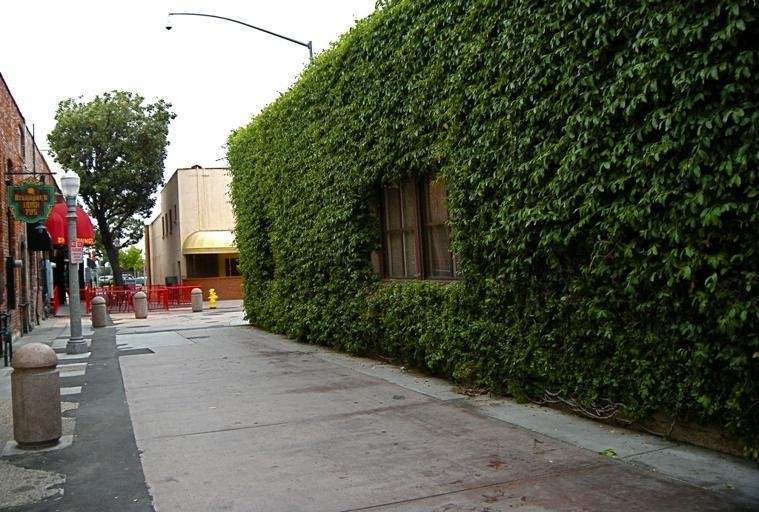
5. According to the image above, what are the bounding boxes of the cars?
[98,273,145,285]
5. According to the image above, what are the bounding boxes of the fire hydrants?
[208,287,218,309]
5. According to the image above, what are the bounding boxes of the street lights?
[60,168,87,354]
